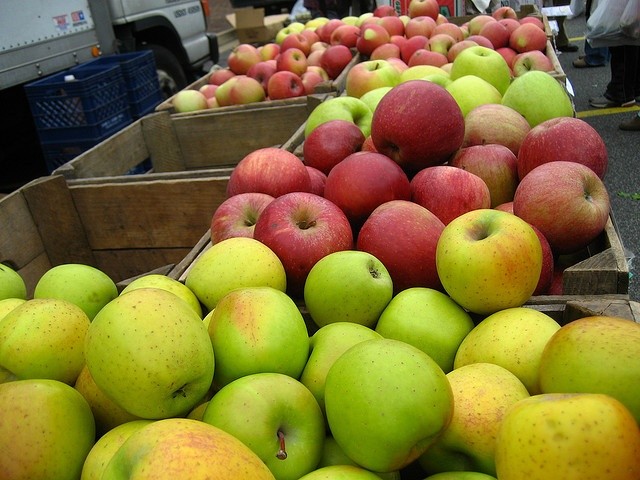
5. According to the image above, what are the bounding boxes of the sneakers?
[579,55,586,59]
[573,59,588,67]
[589,95,636,109]
[619,113,639,130]
[635,96,639,106]
[561,43,578,51]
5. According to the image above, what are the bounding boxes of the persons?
[586,0,640,107]
[618,112,640,130]
[573,0,611,68]
[544,0,579,52]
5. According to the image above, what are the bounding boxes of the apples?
[371,80,465,165]
[370,43,400,60]
[494,47,518,71]
[357,200,446,293]
[325,339,454,473]
[492,6,518,22]
[405,16,437,39]
[424,34,456,59]
[498,18,521,34]
[100,417,276,480]
[346,60,399,98]
[207,286,309,387]
[448,40,480,62]
[0,379,96,480]
[172,13,360,112]
[0,298,91,387]
[81,419,161,480]
[459,27,469,40]
[464,35,494,49]
[33,264,118,322]
[408,48,448,67]
[298,321,387,425]
[253,192,354,285]
[399,65,453,88]
[390,35,408,49]
[201,373,326,480]
[360,87,394,114]
[461,103,532,158]
[211,193,276,246]
[72,363,141,428]
[454,308,562,396]
[511,50,555,76]
[377,16,405,37]
[536,316,640,428]
[409,0,439,21]
[399,15,411,28]
[501,70,574,129]
[373,5,398,18]
[355,23,390,57]
[479,21,510,49]
[303,120,366,176]
[450,46,510,98]
[449,139,517,208]
[430,23,464,43]
[468,15,498,35]
[519,16,544,31]
[225,147,312,200]
[119,274,203,320]
[297,465,384,480]
[514,161,610,252]
[185,236,287,313]
[417,363,531,479]
[375,286,475,375]
[516,117,608,181]
[410,166,491,226]
[0,263,27,301]
[303,250,393,329]
[495,393,640,479]
[305,96,373,139]
[355,13,374,26]
[447,75,503,119]
[509,22,547,54]
[324,151,411,218]
[85,288,215,419]
[401,35,429,64]
[436,209,543,315]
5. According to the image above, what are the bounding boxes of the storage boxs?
[294,89,356,154]
[51,92,336,184]
[177,154,629,303]
[155,48,360,119]
[1,175,232,288]
[446,4,539,27]
[447,14,552,37]
[342,39,566,86]
[290,299,640,325]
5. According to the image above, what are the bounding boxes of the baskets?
[24,49,164,146]
[41,100,167,178]
